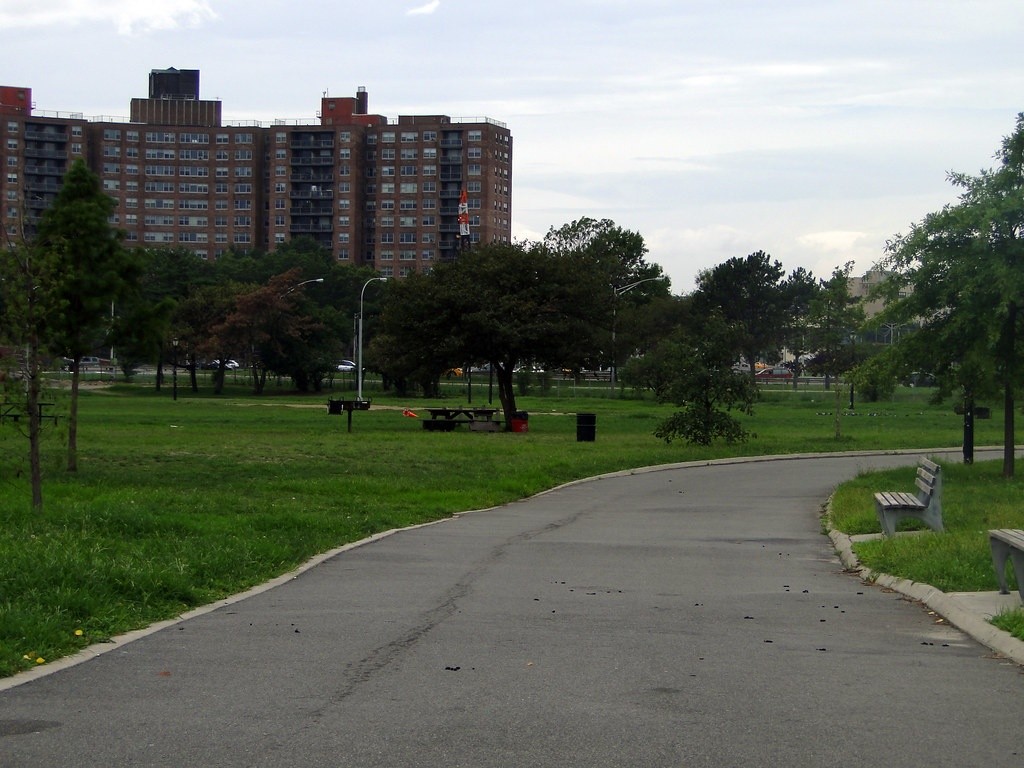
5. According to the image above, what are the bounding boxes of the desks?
[424,408,500,433]
[0,403,55,427]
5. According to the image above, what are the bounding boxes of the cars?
[54,357,100,366]
[444,368,463,380]
[753,366,793,379]
[512,366,544,373]
[201,359,239,370]
[902,371,939,387]
[334,360,366,372]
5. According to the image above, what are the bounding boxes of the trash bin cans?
[509,411,529,433]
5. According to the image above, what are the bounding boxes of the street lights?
[849,330,856,410]
[172,338,179,401]
[610,277,664,398]
[358,277,389,401]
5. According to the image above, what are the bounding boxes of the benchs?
[0,414,22,422]
[874,457,943,541]
[988,529,1024,604]
[422,419,506,424]
[22,415,65,426]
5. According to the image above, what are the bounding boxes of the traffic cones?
[402,408,419,418]
